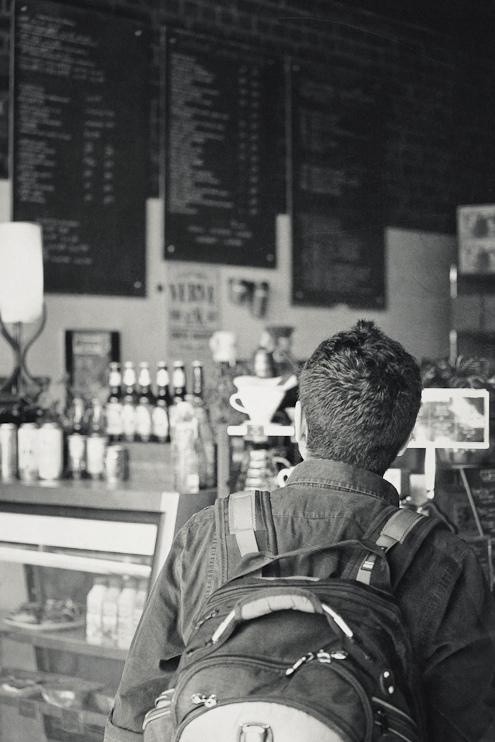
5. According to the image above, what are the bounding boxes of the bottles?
[84,576,150,652]
[0,396,109,485]
[102,360,207,443]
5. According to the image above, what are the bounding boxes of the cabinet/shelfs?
[1,483,218,741]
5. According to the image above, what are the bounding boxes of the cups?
[227,372,298,426]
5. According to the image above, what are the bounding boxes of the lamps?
[0,221,45,388]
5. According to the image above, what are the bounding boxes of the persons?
[102,319,495,742]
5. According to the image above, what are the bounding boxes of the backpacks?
[133,478,457,742]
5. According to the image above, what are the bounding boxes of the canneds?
[0,422,64,482]
[102,444,129,484]
